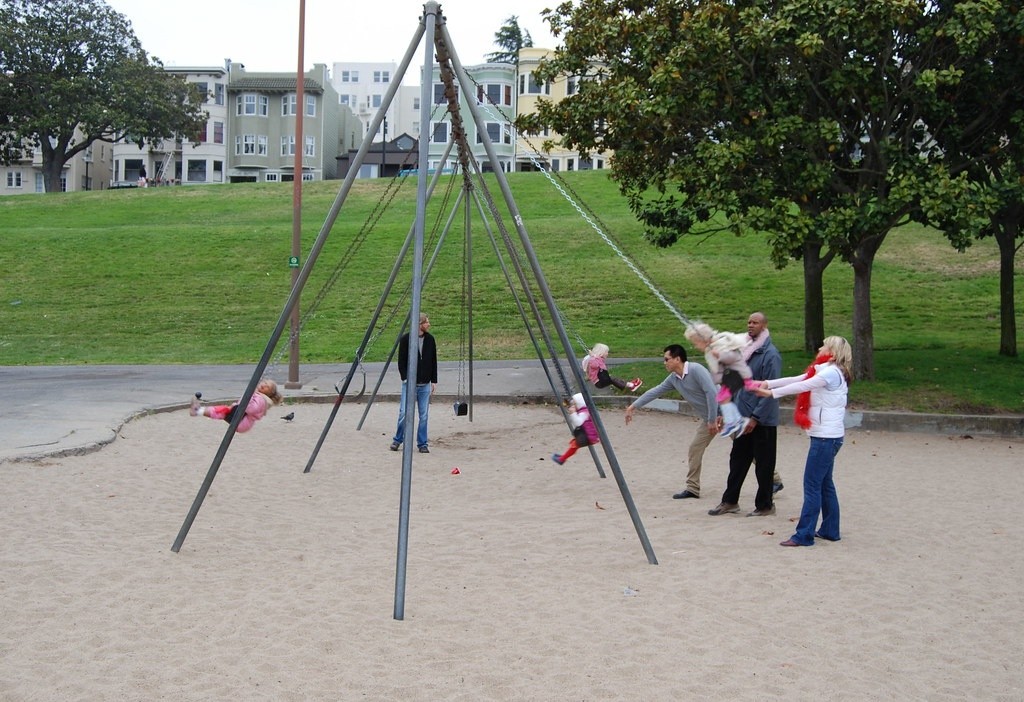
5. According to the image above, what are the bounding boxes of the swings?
[548,282,612,387]
[463,136,592,448]
[334,154,461,401]
[445,57,745,395]
[453,175,468,417]
[223,82,452,425]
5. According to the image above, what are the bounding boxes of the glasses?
[665,356,675,362]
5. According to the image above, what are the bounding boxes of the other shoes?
[553,453,563,465]
[190,396,201,416]
[629,377,642,392]
[673,490,699,499]
[419,446,429,453]
[814,530,817,536]
[390,442,399,450]
[780,540,800,546]
[772,483,784,494]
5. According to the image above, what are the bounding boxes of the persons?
[685,323,752,437]
[708,313,781,516]
[582,343,643,391]
[755,335,852,545]
[552,393,600,465]
[390,313,438,452]
[625,345,784,499]
[190,380,281,433]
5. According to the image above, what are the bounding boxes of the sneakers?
[735,416,750,438]
[747,504,777,518]
[708,501,740,515]
[719,422,739,438]
